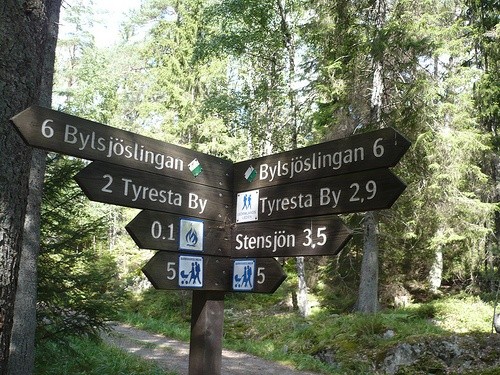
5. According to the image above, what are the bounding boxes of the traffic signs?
[9,105,412,294]
